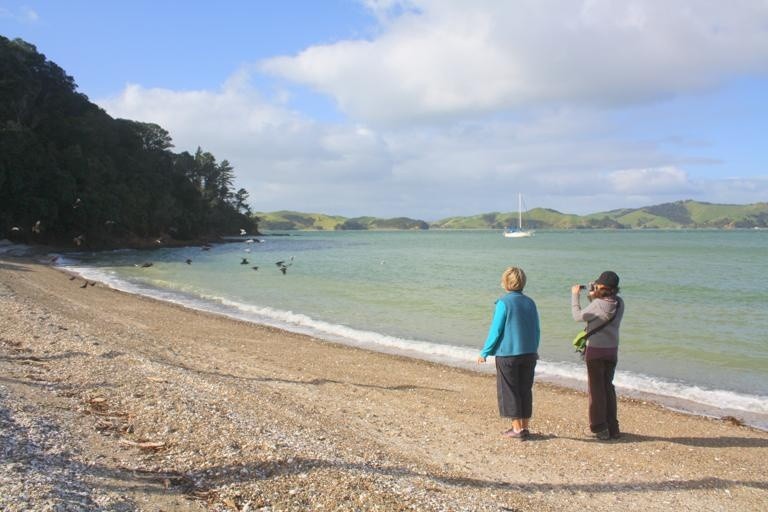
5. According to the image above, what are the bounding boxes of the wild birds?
[80,279,96,288]
[274,258,293,275]
[32,220,41,235]
[185,259,192,265]
[239,227,266,272]
[141,261,154,268]
[69,274,76,281]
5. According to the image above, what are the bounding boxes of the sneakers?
[584,427,620,439]
[500,427,529,440]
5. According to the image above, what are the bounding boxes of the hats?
[595,271,619,287]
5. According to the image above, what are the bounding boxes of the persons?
[571,270,625,440]
[477,266,540,441]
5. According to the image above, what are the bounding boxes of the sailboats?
[504,191,536,238]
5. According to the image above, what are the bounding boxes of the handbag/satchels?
[573,328,586,354]
